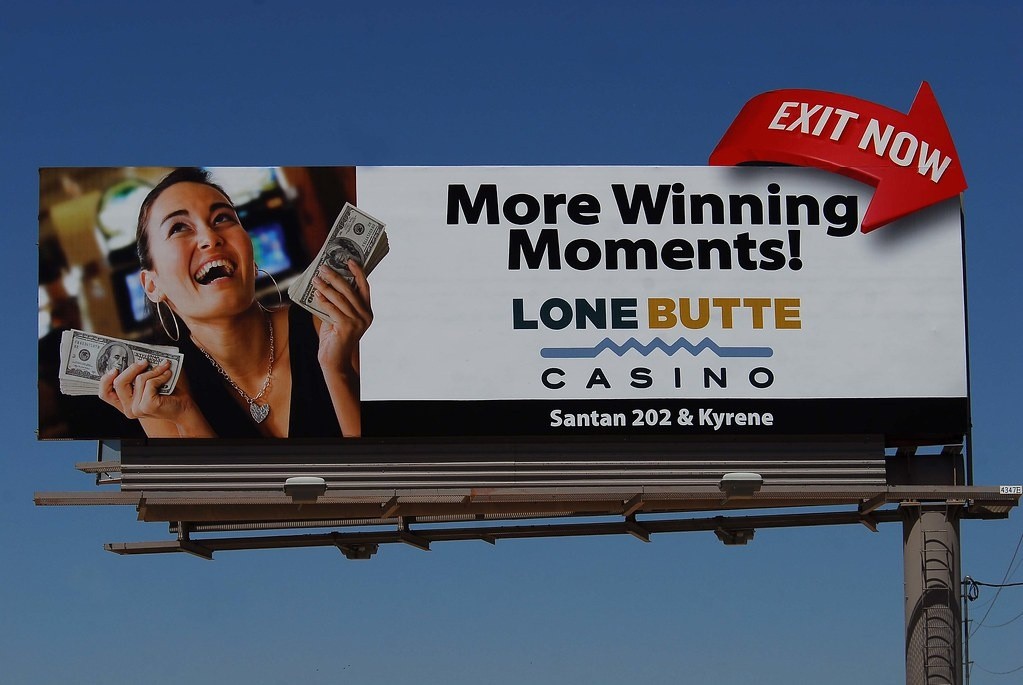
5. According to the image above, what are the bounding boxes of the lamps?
[717,472,765,496]
[281,477,327,504]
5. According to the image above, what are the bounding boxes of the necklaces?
[190,307,274,424]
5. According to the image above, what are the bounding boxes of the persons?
[97,167,372,438]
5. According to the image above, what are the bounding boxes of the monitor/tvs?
[246,225,291,280]
[126,268,153,321]
